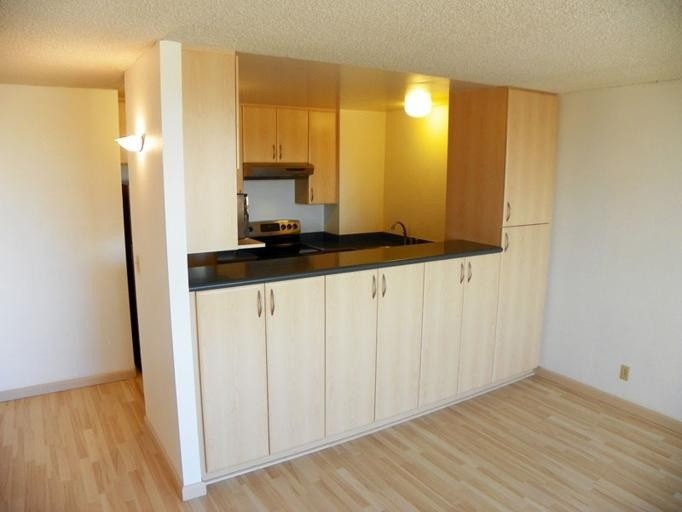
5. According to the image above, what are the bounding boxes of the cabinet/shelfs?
[295,111,337,205]
[444,86,558,385]
[419,251,499,418]
[325,261,426,442]
[190,275,325,480]
[241,103,310,164]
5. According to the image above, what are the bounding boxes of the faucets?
[391,219,408,244]
